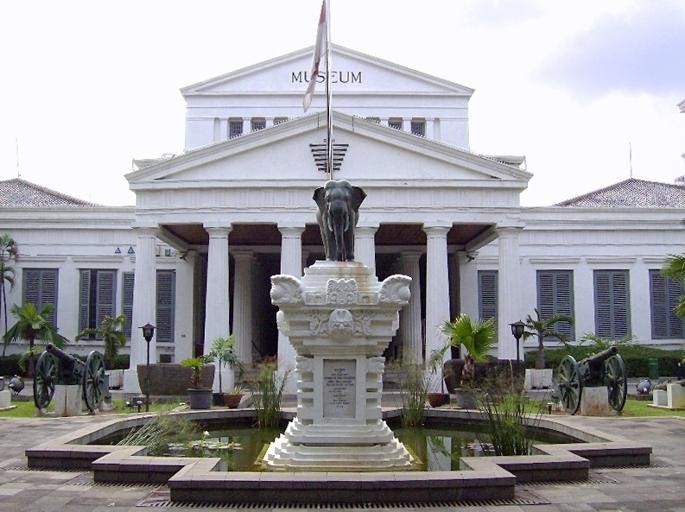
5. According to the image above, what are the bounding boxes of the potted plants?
[439,313,500,409]
[223,386,244,408]
[178,358,212,409]
[521,308,576,388]
[428,345,449,409]
[199,333,247,405]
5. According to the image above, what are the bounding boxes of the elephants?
[312,180,367,261]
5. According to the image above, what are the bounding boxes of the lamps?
[138,323,162,366]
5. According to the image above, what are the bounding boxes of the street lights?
[137,323,156,411]
[509,320,526,416]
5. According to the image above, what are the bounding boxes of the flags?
[302,0,328,114]
